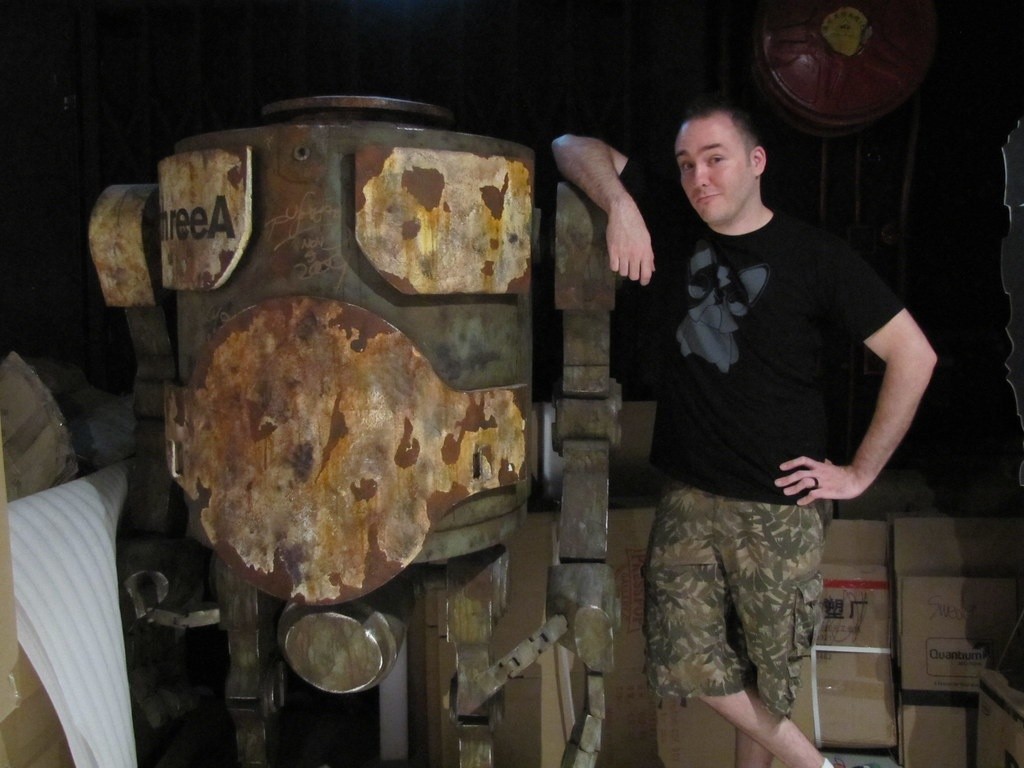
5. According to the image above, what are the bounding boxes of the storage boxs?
[401,397,1024,768]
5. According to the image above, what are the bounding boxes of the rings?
[812,477,818,489]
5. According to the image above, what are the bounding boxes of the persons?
[551,96,938,768]
